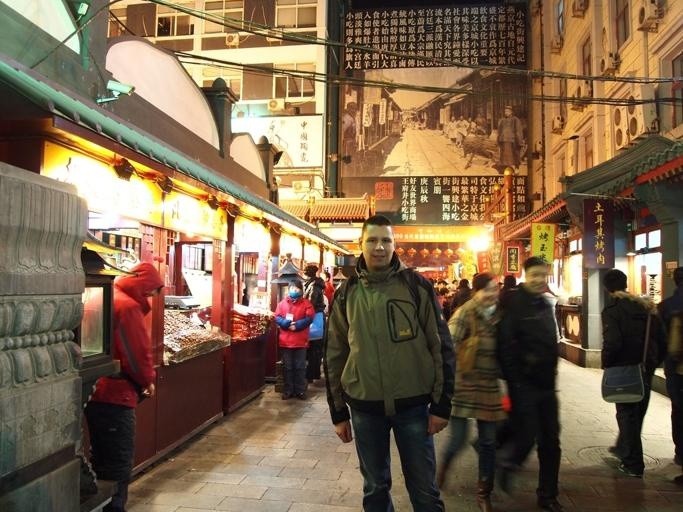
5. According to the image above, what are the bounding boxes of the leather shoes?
[536,495,568,512]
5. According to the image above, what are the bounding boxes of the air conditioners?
[292,180,312,194]
[265,28,283,42]
[534,0,662,153]
[226,33,240,46]
[267,98,285,112]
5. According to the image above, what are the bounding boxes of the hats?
[303,265,318,278]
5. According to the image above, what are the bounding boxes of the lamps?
[626,246,649,257]
[112,157,344,256]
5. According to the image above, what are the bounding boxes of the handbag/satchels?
[599,363,647,402]
[456,337,476,371]
[307,313,324,341]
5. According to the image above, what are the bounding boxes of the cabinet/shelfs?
[79,307,280,479]
[554,305,584,344]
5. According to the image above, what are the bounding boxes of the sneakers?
[280,375,322,401]
[608,444,682,482]
[496,473,513,495]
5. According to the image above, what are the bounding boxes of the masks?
[286,291,302,298]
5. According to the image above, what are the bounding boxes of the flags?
[525,222,557,278]
[499,241,523,277]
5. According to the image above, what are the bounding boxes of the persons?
[435,272,508,512]
[493,103,524,172]
[599,269,666,479]
[274,263,562,402]
[439,111,491,147]
[84,262,166,512]
[80,246,104,278]
[657,267,683,484]
[322,216,455,512]
[498,257,568,511]
[341,100,361,175]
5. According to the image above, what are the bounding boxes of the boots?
[437,450,451,487]
[474,479,494,511]
